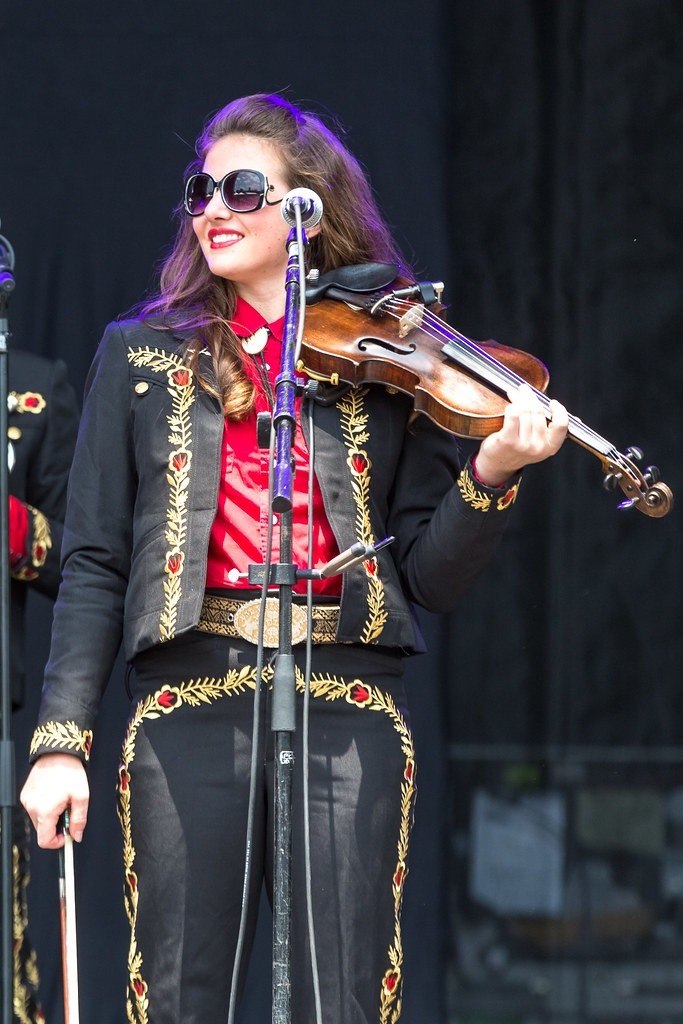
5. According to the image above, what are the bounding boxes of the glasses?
[183,169,282,217]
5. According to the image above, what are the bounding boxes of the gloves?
[8,495,29,565]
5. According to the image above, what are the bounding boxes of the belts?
[192,596,355,648]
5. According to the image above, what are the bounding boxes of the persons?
[20,94,570,1023]
[0,239,81,1024]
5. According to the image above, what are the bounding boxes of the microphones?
[282,187,323,228]
[0,245,16,291]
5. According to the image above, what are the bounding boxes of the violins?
[289,260,674,519]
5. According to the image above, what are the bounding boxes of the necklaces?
[239,324,300,439]
